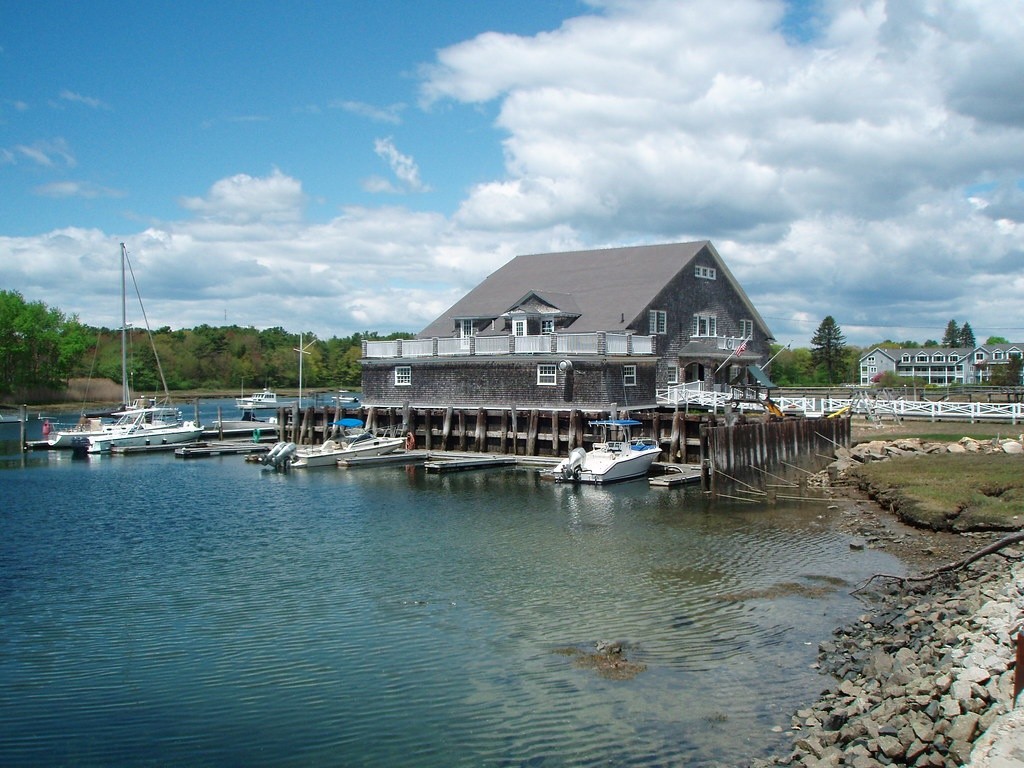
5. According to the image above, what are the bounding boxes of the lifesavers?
[406,432,415,450]
[252,429,261,442]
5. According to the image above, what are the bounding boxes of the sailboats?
[42,240,207,455]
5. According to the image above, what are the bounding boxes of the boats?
[261,427,409,469]
[234,371,297,410]
[331,394,356,401]
[550,417,664,486]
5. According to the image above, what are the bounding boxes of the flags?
[734,337,748,357]
